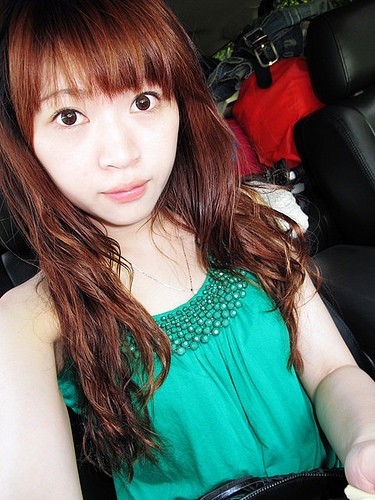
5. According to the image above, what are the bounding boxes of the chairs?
[296,1,375,257]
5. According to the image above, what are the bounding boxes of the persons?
[1,0,375,500]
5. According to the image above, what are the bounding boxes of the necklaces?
[122,227,195,294]
